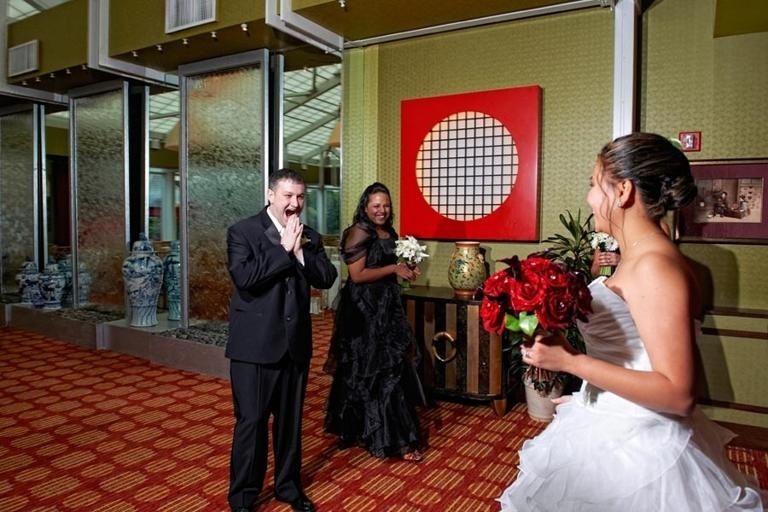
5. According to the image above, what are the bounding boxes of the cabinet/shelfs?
[401,285,525,419]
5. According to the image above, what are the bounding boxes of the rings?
[525,348,531,359]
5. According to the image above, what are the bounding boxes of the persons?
[223,168,337,512]
[590,219,672,277]
[490,131,768,512]
[323,182,433,462]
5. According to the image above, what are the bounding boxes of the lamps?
[164,120,181,151]
[328,117,341,148]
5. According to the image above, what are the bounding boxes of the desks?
[309,244,340,315]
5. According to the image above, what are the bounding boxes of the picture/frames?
[668,157,767,248]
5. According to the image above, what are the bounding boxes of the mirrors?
[37,97,78,310]
[142,77,187,330]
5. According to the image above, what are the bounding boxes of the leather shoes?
[275,489,316,512]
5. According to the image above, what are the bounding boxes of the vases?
[447,240,488,297]
[14,259,42,305]
[520,375,564,425]
[37,262,67,310]
[163,240,184,322]
[122,236,165,328]
[62,255,90,307]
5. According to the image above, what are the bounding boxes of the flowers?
[477,205,594,399]
[392,235,429,288]
[589,229,620,279]
